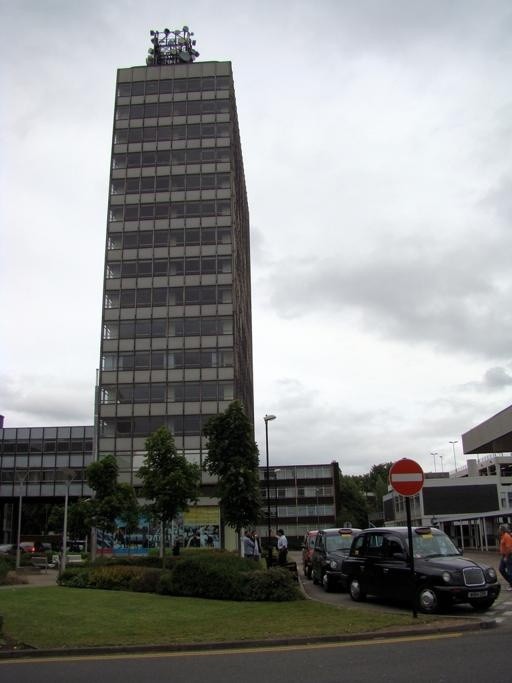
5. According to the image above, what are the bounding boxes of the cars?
[0,539,89,554]
[97,528,218,550]
[301,524,501,613]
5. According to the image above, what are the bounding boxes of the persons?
[277,528,288,565]
[497,523,512,591]
[250,529,262,559]
[243,530,253,558]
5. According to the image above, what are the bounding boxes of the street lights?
[240,468,281,560]
[13,466,31,568]
[439,454,444,471]
[430,452,437,472]
[263,413,277,568]
[59,467,79,575]
[448,439,458,473]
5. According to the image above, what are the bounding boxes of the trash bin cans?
[281,562,299,583]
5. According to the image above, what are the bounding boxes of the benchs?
[31,555,82,574]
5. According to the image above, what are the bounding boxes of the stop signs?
[388,457,424,495]
